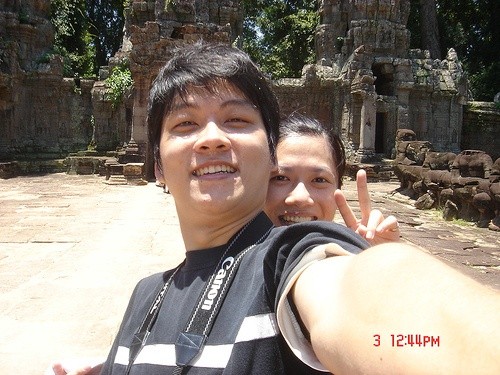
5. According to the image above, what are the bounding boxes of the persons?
[52,115,400,375]
[97,36,500,375]
